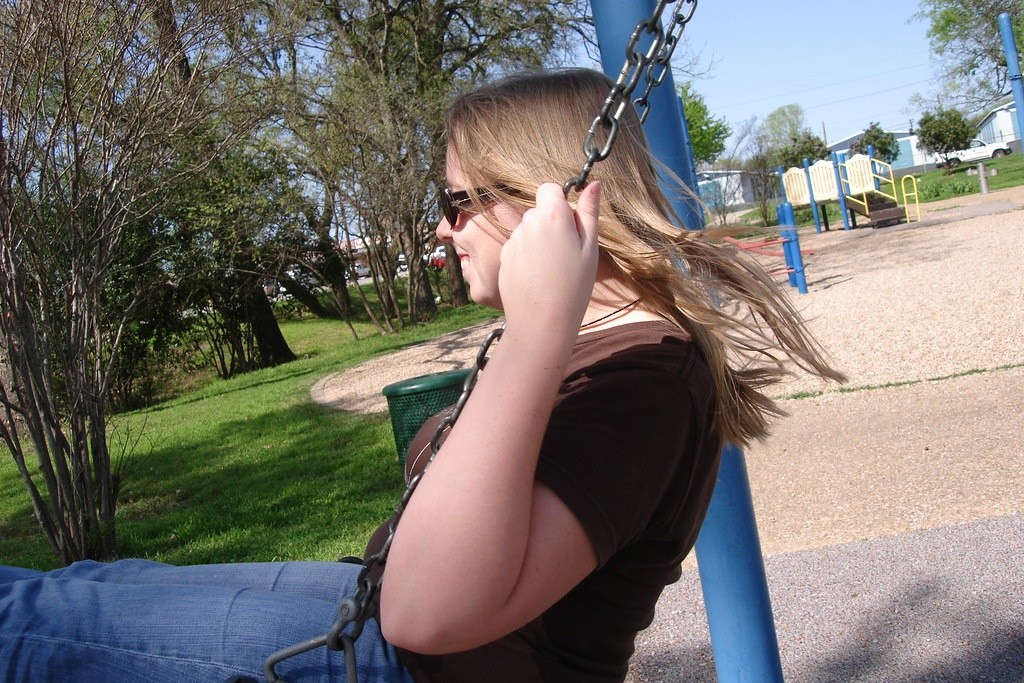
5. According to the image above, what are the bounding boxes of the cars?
[263,270,310,295]
[397,255,409,273]
[431,254,446,270]
[934,136,1012,170]
[345,263,371,280]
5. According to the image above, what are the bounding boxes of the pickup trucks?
[423,246,445,265]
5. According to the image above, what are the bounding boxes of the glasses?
[439,184,520,230]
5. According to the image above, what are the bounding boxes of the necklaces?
[580,294,646,329]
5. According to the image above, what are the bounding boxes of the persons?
[0,68,848,683]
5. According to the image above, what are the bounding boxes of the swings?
[260,0,700,683]
